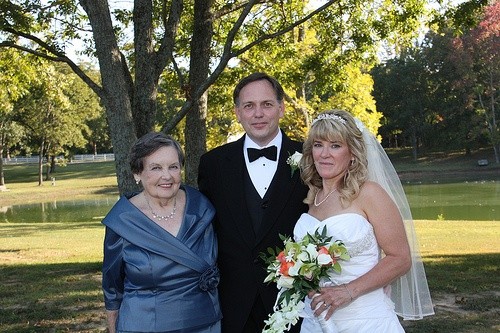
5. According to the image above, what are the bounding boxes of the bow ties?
[246,145,278,163]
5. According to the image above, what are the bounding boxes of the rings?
[324,302,327,309]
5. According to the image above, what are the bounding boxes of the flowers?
[287,151,303,177]
[259,225,351,333]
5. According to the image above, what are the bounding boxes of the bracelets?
[343,284,353,300]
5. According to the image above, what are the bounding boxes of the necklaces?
[144,194,177,221]
[314,187,338,207]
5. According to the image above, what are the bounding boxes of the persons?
[198,73,323,333]
[293,110,435,333]
[102,132,222,333]
